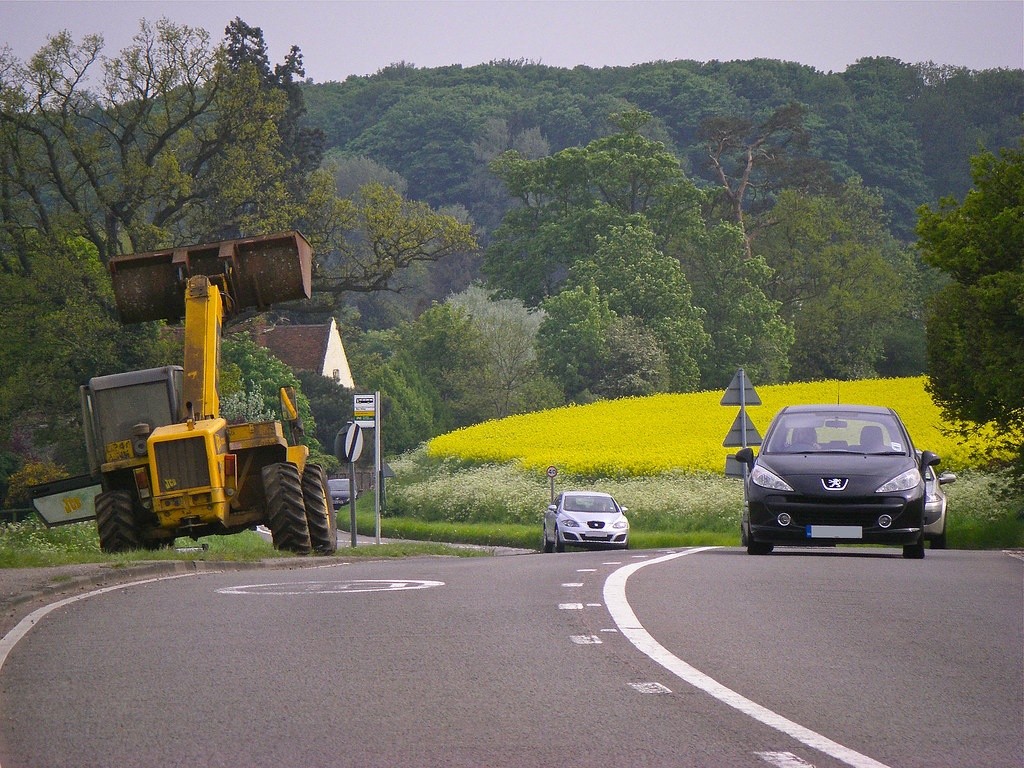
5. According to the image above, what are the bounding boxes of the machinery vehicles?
[30,229,337,558]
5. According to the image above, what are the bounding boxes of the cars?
[328,478,363,510]
[543,491,630,553]
[913,449,957,550]
[736,404,941,560]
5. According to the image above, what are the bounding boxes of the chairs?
[860,425,883,445]
[594,497,603,504]
[791,428,818,444]
[567,499,577,507]
